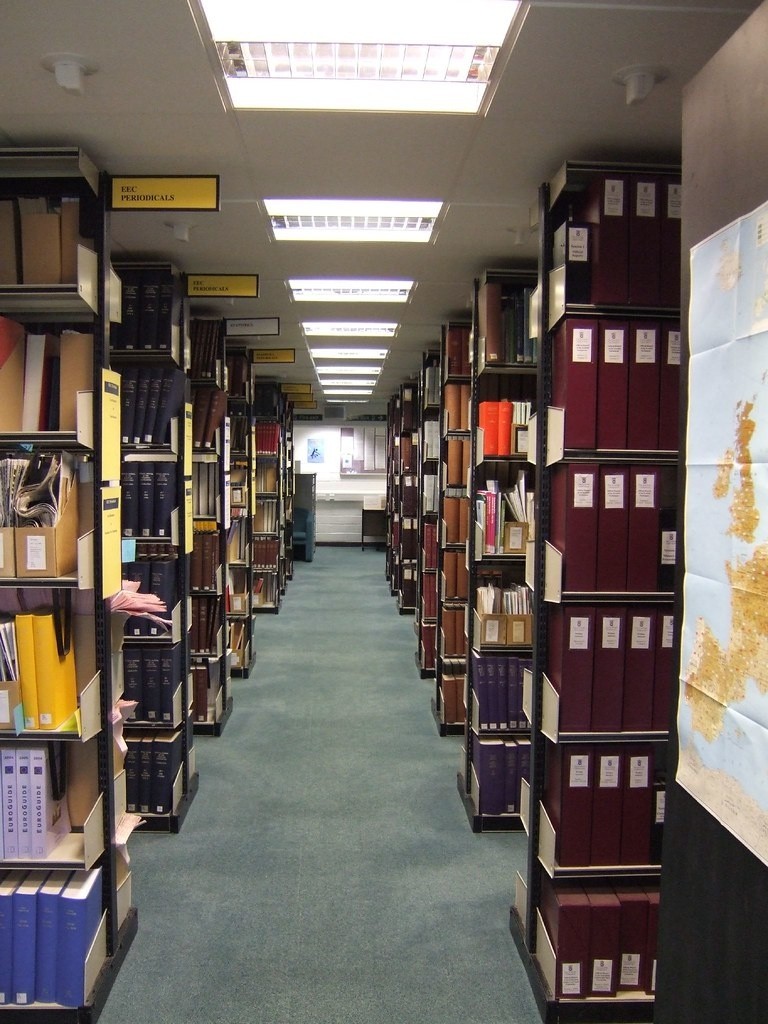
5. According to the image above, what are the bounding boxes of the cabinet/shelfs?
[0,145,294,1024]
[387,96,680,1024]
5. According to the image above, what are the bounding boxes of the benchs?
[293,512,315,562]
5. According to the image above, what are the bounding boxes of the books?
[107,268,196,816]
[387,352,441,677]
[533,176,684,1000]
[189,321,230,722]
[227,349,295,669]
[464,279,536,817]
[438,326,470,725]
[0,194,107,1008]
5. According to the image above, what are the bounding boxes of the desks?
[362,509,388,551]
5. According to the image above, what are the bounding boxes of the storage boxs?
[363,495,386,510]
[16,463,78,578]
